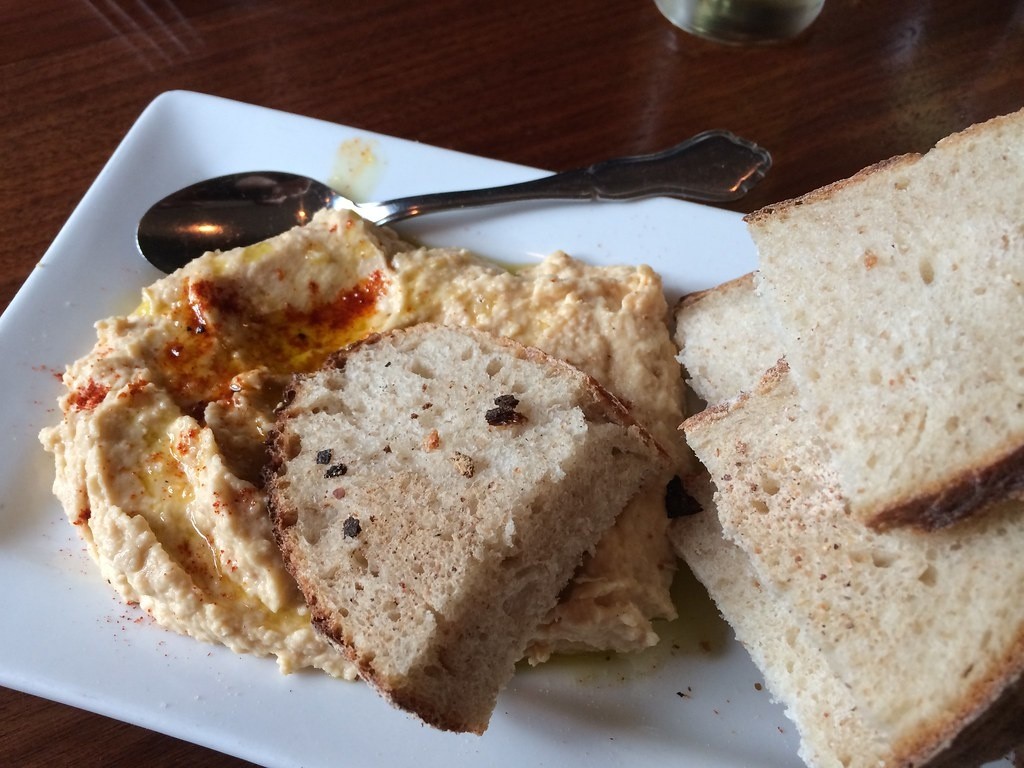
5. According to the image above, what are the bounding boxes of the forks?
[85,0,208,73]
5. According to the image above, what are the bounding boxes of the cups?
[653,0,825,45]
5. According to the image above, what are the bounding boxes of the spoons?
[134,129,772,277]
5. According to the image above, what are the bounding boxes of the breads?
[256,109,1024,768]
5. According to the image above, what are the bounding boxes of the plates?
[0,89,1024,768]
